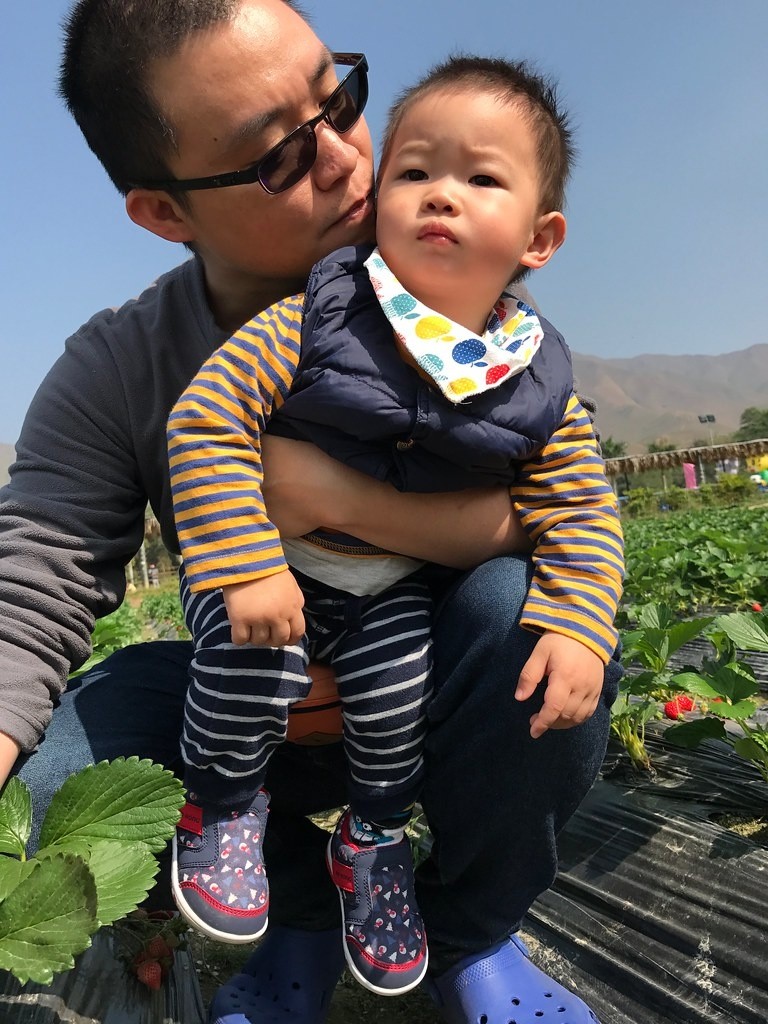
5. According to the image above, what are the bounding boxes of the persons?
[161,56,624,995]
[0,0,616,1024]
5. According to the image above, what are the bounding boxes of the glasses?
[157,49,369,201]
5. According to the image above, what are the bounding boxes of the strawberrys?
[670,591,764,616]
[124,601,192,644]
[642,682,735,725]
[119,905,179,989]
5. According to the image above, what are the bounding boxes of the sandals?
[424,926,600,1024]
[202,924,351,1023]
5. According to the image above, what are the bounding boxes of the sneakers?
[173,783,271,946]
[326,808,430,992]
